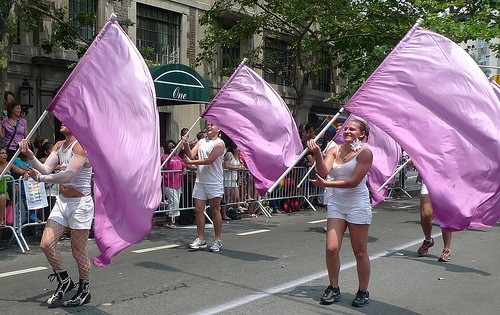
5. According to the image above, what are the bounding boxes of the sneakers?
[417,238,435,256]
[438,248,452,262]
[188,237,207,248]
[322,286,341,306]
[351,289,370,307]
[209,240,223,253]
[45,269,92,308]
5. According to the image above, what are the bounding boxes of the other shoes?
[164,223,178,229]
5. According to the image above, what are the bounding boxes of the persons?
[306,119,372,308]
[17,123,94,307]
[181,122,226,252]
[416,178,452,262]
[0,91,94,250]
[487,75,500,98]
[161,124,337,229]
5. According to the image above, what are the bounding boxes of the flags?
[47,17,162,269]
[344,24,500,231]
[321,115,339,135]
[333,112,401,208]
[199,63,304,198]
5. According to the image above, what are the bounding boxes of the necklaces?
[60,139,76,152]
[339,145,353,161]
